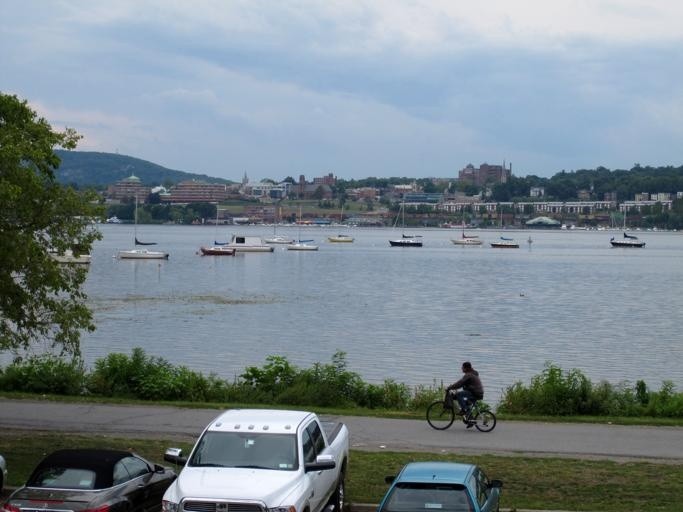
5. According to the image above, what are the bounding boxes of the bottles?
[463,362,471,368]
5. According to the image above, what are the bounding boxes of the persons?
[445,361,483,428]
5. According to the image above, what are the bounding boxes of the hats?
[378,462,504,511]
[1,449,177,512]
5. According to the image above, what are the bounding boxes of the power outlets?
[163,408,349,512]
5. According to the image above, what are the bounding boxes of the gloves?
[202,234,274,256]
[119,250,169,259]
[266,235,318,251]
[610,233,645,247]
[451,234,520,248]
[327,236,353,242]
[48,248,91,264]
[390,235,424,247]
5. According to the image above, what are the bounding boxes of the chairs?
[467,422,474,428]
[456,410,468,415]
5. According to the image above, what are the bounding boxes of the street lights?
[443,391,453,409]
